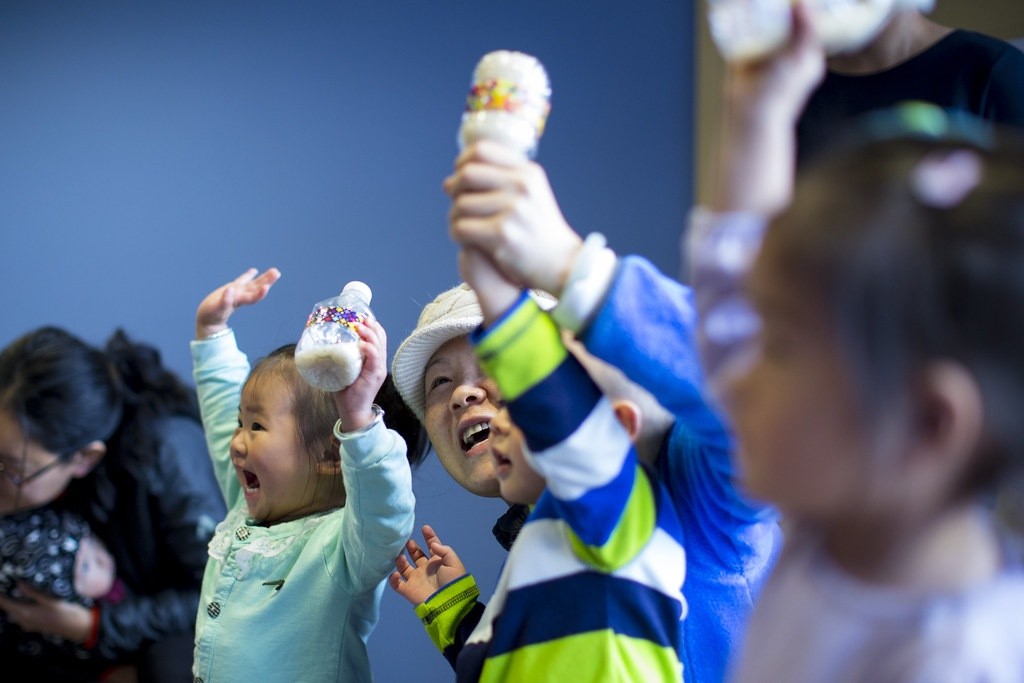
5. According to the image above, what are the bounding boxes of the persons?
[390,141,786,682]
[681,2,1024,682]
[190,265,432,683]
[0,325,228,683]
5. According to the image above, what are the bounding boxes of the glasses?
[0,444,86,498]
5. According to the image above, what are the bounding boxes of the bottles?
[456,49,550,163]
[704,0,938,70]
[293,281,372,391]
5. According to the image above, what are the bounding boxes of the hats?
[392,280,562,427]
